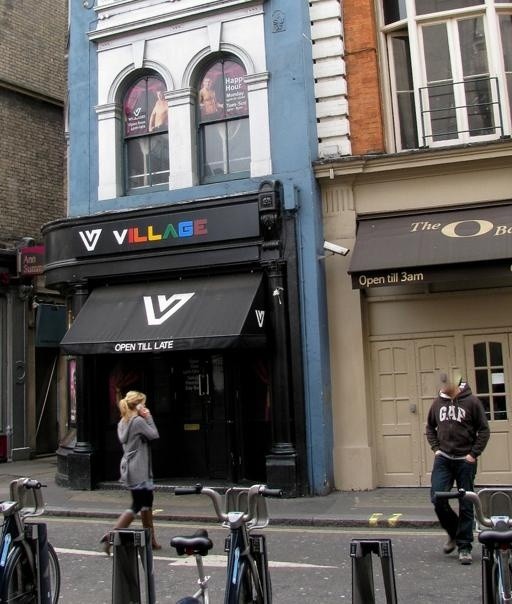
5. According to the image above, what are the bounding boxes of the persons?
[148,87,168,132]
[97,389,162,557]
[425,369,491,565]
[198,78,224,120]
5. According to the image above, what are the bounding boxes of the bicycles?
[168,484,282,603]
[431,487,512,603]
[0,478,61,604]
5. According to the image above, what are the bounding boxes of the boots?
[141,510,161,551]
[99,510,135,557]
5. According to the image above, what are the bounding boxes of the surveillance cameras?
[323,241,350,256]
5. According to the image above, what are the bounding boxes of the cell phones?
[136,403,143,411]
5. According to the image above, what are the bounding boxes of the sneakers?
[458,551,473,565]
[443,535,457,555]
